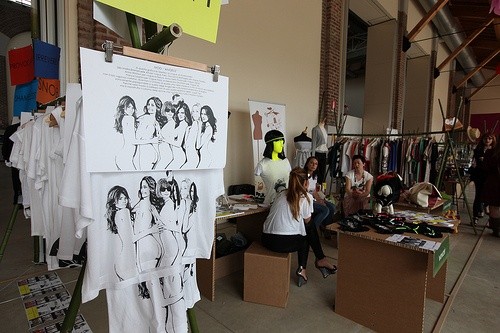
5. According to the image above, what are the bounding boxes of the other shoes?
[478,211,485,218]
[483,207,490,216]
[471,216,480,226]
[489,229,500,239]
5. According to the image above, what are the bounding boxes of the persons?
[292,129,314,170]
[469,132,496,224]
[300,157,338,231]
[312,116,330,185]
[339,155,373,216]
[252,128,293,207]
[259,167,338,287]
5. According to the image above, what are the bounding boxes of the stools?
[244,241,292,308]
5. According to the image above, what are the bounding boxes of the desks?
[326,207,449,333]
[195,197,273,301]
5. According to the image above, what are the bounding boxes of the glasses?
[483,137,492,140]
[160,186,172,192]
[165,108,175,113]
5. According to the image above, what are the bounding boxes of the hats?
[467,126,480,142]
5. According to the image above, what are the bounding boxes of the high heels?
[316,264,339,279]
[296,265,309,287]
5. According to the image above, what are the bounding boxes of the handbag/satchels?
[373,171,401,205]
[466,164,476,183]
[407,181,442,207]
